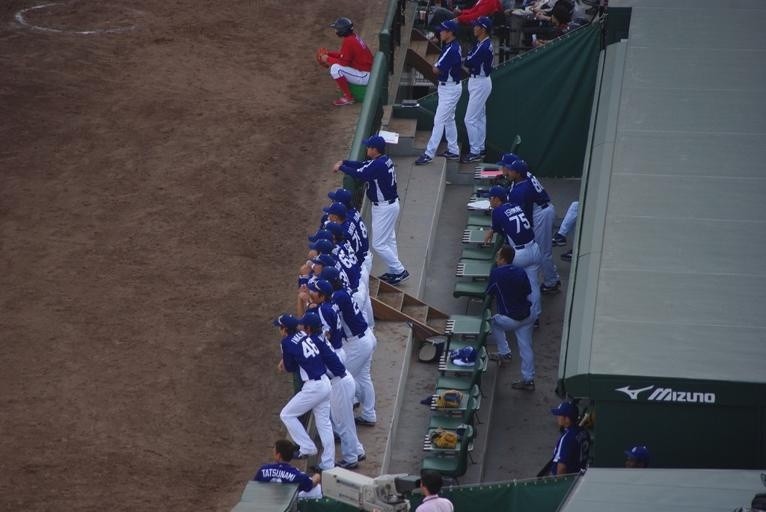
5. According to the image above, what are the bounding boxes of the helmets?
[328,16,354,37]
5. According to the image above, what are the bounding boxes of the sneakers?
[560,249,572,261]
[512,378,535,391]
[487,352,512,362]
[533,318,541,328]
[330,94,356,107]
[552,232,568,247]
[414,153,432,165]
[538,282,562,291]
[437,151,460,160]
[461,149,488,163]
[292,403,379,476]
[379,269,411,285]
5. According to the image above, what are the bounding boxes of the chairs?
[419,133,523,486]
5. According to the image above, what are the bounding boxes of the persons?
[252,439,322,499]
[314,16,373,106]
[549,397,586,477]
[414,1,574,166]
[412,468,455,512]
[332,136,409,286]
[272,314,335,470]
[621,445,648,469]
[298,189,378,424]
[481,150,581,393]
[297,315,365,467]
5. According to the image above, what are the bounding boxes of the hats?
[434,20,458,32]
[297,312,323,327]
[272,313,297,329]
[361,134,386,148]
[623,442,650,461]
[548,400,580,420]
[305,187,355,298]
[495,153,529,174]
[469,15,494,32]
[448,344,477,364]
[481,185,508,198]
